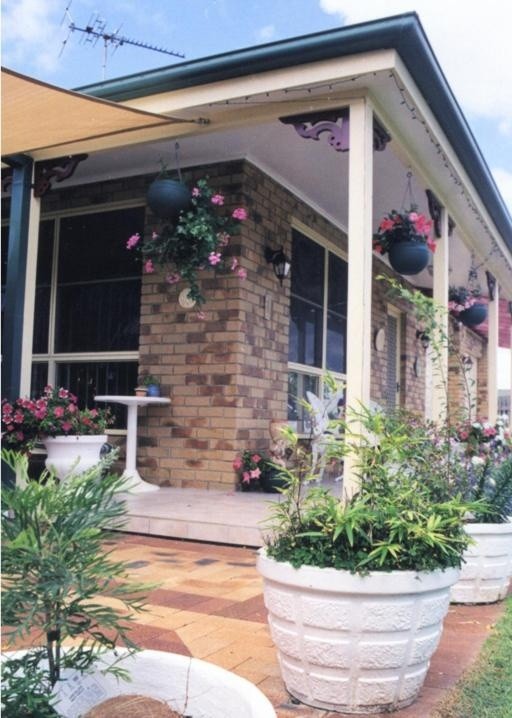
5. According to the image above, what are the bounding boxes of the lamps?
[264,244,293,287]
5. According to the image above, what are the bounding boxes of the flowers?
[1,384,116,453]
[372,203,436,256]
[125,173,247,319]
[233,439,285,485]
[448,283,488,312]
[410,412,511,522]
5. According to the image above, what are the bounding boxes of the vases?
[241,469,289,494]
[134,388,149,396]
[42,434,108,499]
[450,515,511,606]
[146,179,192,221]
[388,242,430,276]
[459,303,486,327]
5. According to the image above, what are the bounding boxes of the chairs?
[302,390,344,486]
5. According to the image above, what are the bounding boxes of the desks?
[295,433,344,482]
[94,395,172,493]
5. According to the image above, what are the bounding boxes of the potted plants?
[144,375,161,397]
[254,368,496,714]
